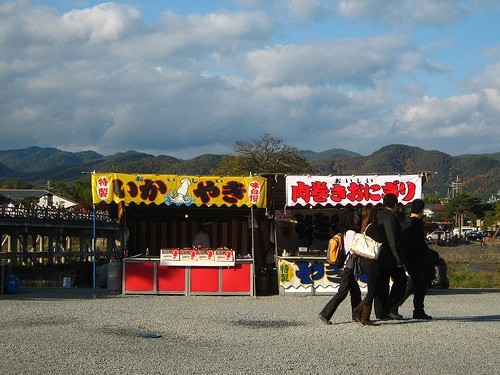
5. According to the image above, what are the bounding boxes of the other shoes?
[413,310,432,321]
[388,312,403,321]
[318,314,332,325]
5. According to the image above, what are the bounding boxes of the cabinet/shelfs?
[123,254,254,296]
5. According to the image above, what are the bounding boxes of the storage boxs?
[299,247,308,251]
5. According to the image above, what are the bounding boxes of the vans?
[426,231,446,241]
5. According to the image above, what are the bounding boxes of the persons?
[192,220,211,247]
[318,194,432,325]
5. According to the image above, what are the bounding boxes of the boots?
[360,306,374,325]
[353,301,367,322]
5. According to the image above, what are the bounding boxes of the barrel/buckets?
[5,274,19,294]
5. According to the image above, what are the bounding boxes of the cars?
[453,227,486,242]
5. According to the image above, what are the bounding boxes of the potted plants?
[21,195,39,218]
[73,201,119,222]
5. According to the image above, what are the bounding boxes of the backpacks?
[326,232,348,269]
[422,256,449,290]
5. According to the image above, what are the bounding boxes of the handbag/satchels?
[350,223,382,260]
